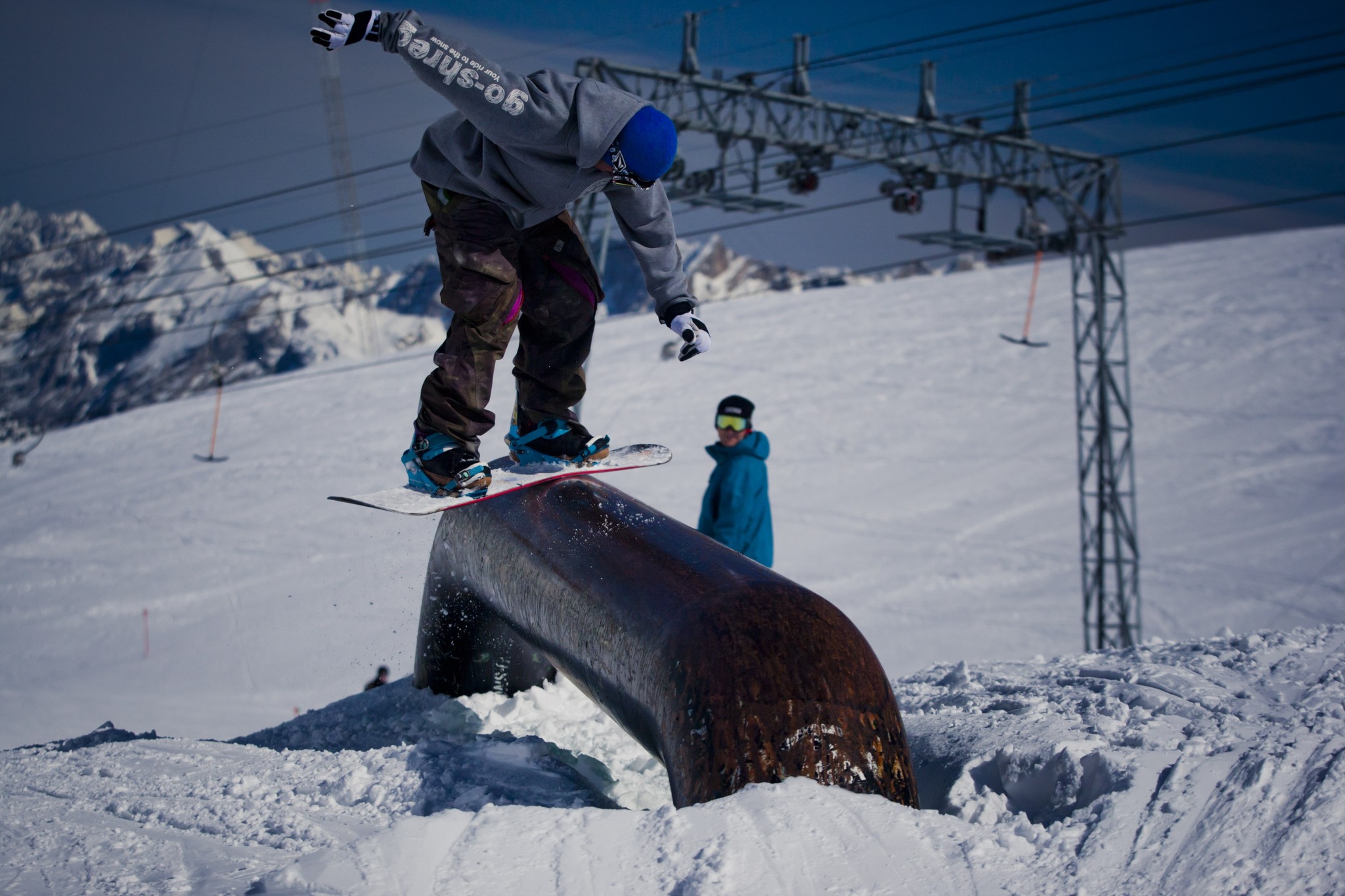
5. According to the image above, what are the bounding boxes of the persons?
[311,8,713,490]
[697,394,775,570]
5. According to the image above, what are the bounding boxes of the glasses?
[716,415,747,432]
[613,174,637,187]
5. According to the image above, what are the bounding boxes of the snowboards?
[325,442,673,517]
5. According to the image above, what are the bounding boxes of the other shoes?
[400,442,493,491]
[507,423,611,463]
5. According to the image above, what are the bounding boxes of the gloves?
[310,7,381,52]
[664,301,711,362]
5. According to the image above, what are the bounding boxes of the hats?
[604,106,679,180]
[719,395,755,421]
